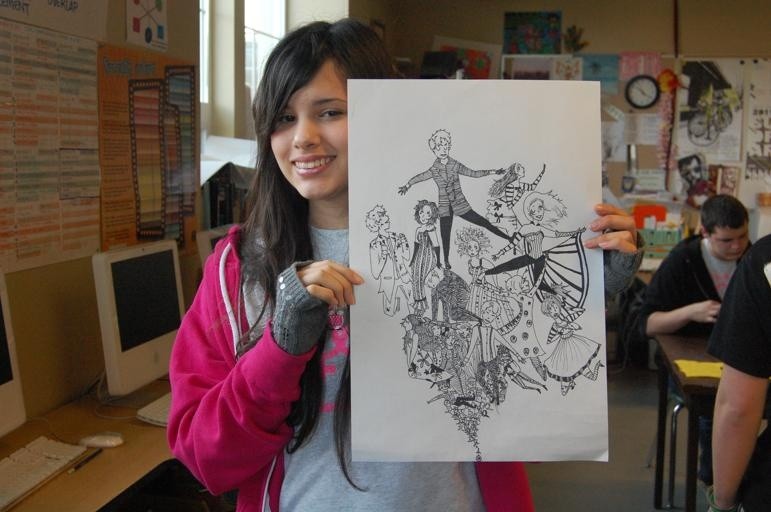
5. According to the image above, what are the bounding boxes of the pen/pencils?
[68,448,102,473]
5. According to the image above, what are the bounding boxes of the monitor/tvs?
[0,266,27,438]
[93,241,186,409]
[196,223,237,272]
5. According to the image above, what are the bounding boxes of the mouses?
[78,434,123,448]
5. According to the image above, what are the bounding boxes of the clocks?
[625,74,658,109]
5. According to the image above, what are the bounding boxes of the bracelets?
[706,486,740,511]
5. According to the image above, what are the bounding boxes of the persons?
[630,191,751,336]
[700,236,770,510]
[678,155,705,190]
[167,17,646,511]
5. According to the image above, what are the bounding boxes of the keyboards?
[136,390,172,427]
[0,435,87,512]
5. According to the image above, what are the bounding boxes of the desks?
[2,377,175,510]
[650,332,727,510]
